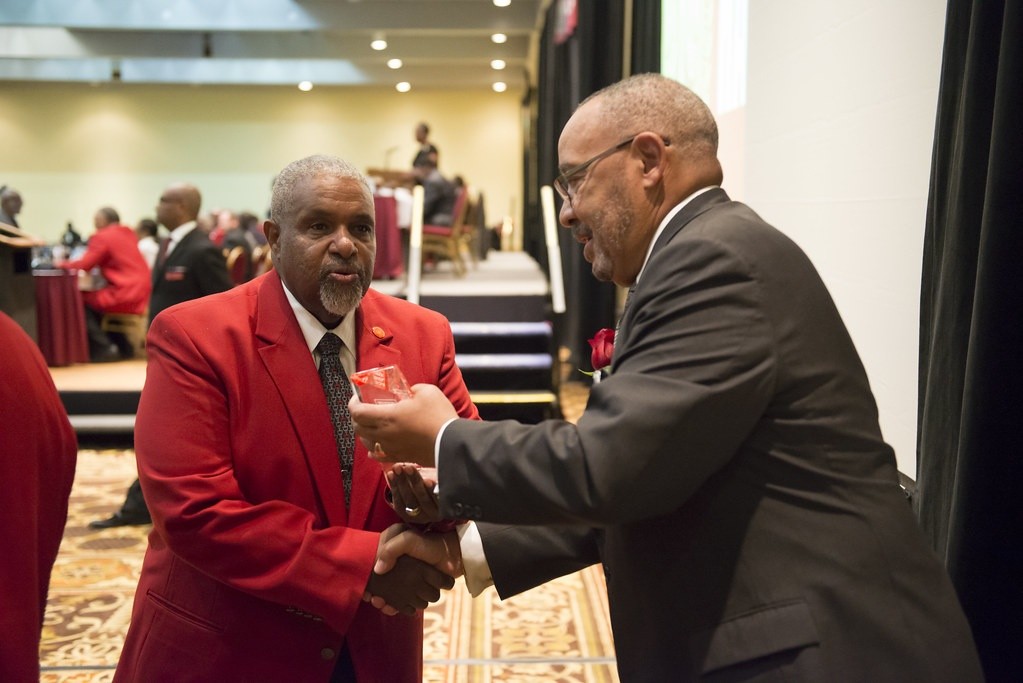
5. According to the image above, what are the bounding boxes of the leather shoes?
[89,515,153,528]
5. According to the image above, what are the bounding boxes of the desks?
[372,195,404,282]
[34,268,91,369]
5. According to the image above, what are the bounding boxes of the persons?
[106,152,482,683]
[0,311,79,683]
[89,182,233,531]
[410,120,439,186]
[135,218,159,270]
[347,72,985,683]
[418,153,456,273]
[0,185,275,344]
[54,206,152,363]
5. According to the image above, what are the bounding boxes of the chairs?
[100,237,173,353]
[409,183,469,280]
[462,191,490,262]
[224,244,274,289]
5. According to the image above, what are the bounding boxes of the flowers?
[576,328,615,382]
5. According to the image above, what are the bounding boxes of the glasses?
[553,134,670,207]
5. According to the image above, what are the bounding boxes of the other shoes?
[92,344,122,361]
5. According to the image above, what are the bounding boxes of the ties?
[316,333,355,515]
[152,238,173,288]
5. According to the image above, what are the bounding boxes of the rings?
[404,506,421,517]
